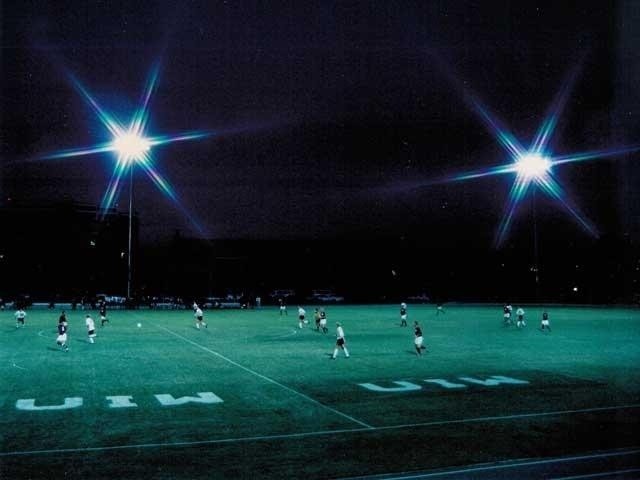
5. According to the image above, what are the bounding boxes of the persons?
[14,308,26,328]
[436,299,445,316]
[279,298,287,315]
[120,295,183,310]
[313,308,328,334]
[414,321,427,356]
[331,322,350,360]
[195,292,256,309]
[400,303,408,327]
[503,302,526,328]
[49,296,109,349]
[193,302,208,329]
[297,306,308,328]
[542,310,550,331]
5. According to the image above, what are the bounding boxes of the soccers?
[137,323,140,327]
